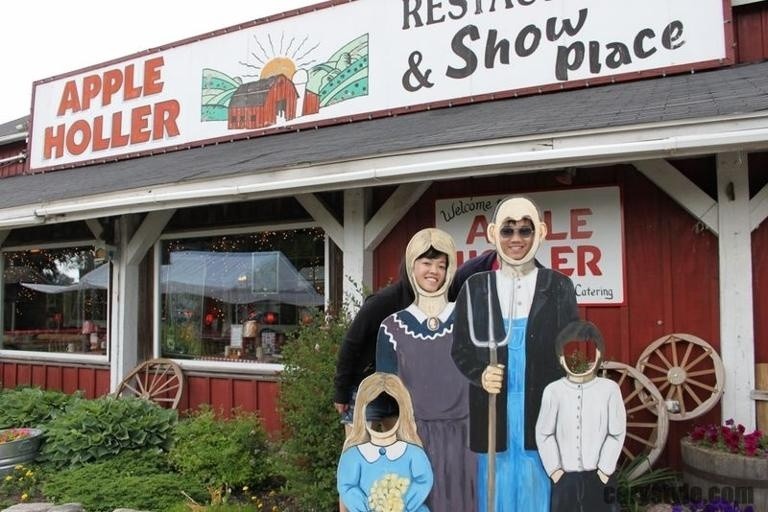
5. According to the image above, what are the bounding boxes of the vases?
[681,434,768,512]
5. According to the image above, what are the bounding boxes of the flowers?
[0,426,31,444]
[688,418,767,458]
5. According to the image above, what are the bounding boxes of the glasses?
[501,228,531,237]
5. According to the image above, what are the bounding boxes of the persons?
[373,226,476,510]
[450,195,579,510]
[448,217,546,303]
[534,317,627,511]
[335,371,435,511]
[336,241,448,430]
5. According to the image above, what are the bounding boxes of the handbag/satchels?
[340,386,382,422]
